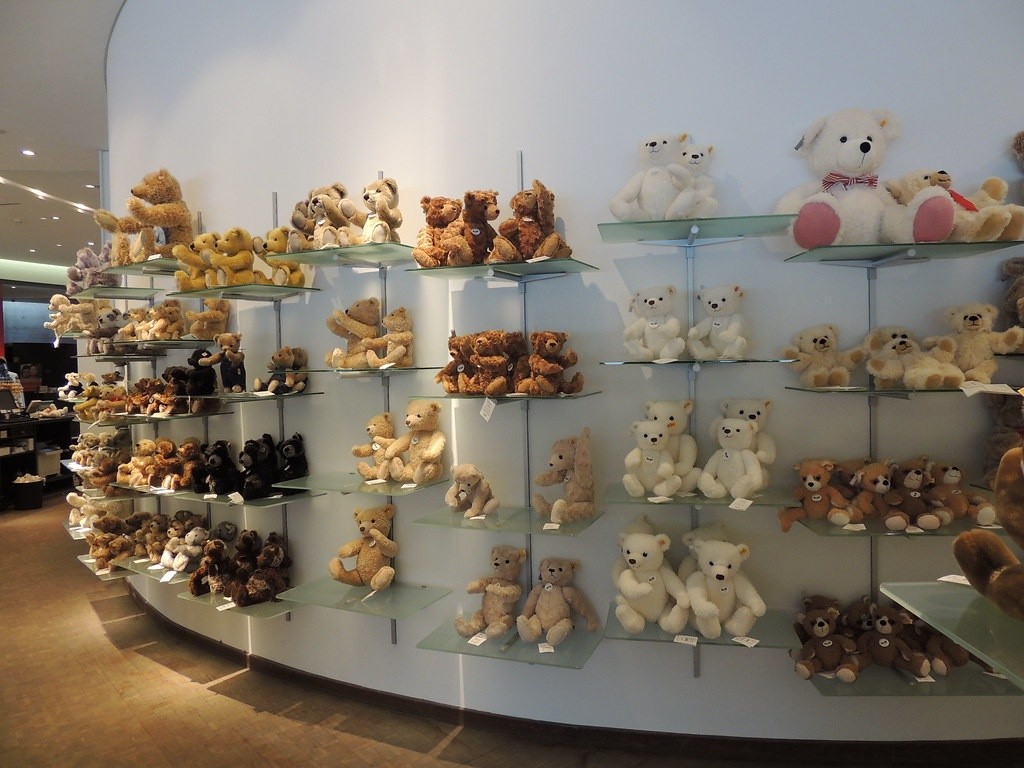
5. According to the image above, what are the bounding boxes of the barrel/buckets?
[14,480,44,509]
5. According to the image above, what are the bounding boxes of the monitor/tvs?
[0,388,18,410]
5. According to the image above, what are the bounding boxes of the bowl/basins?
[32,387,39,392]
[48,388,57,393]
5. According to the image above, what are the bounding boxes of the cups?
[39,386,48,393]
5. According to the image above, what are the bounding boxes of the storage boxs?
[39,446,64,478]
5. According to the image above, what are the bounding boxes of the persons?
[23,365,40,378]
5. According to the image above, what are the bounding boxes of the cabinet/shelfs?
[55,204,1024,718]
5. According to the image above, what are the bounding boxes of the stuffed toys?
[623,283,752,359]
[43,167,230,341]
[985,394,1024,489]
[38,332,310,603]
[611,513,766,639]
[622,397,776,498]
[325,296,414,369]
[774,110,1024,249]
[953,444,1024,620]
[776,455,996,534]
[790,594,1002,684]
[433,329,584,396]
[172,225,305,291]
[289,178,403,253]
[413,178,572,267]
[609,132,718,222]
[784,257,1024,389]
[329,400,596,645]
